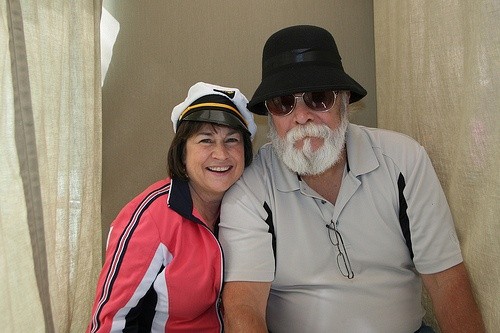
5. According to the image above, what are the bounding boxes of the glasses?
[326,220,355,281]
[265,90,340,116]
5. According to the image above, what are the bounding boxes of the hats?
[246,25,368,116]
[171,81,257,143]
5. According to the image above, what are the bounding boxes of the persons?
[218,23,489,333]
[86,82,257,333]
